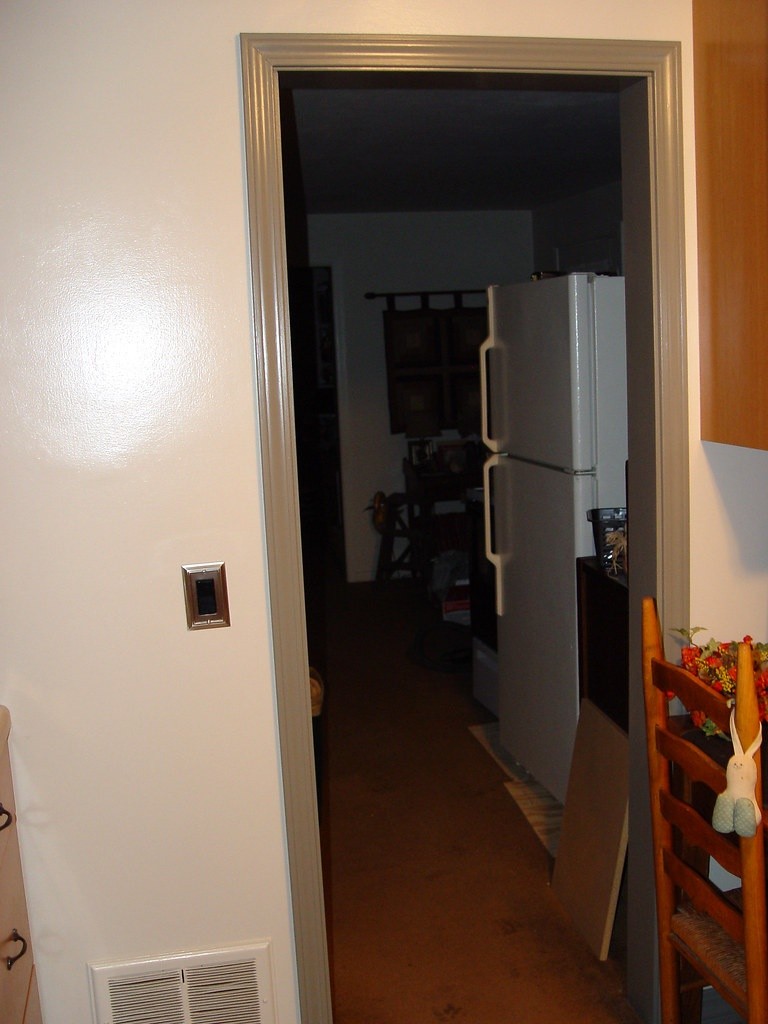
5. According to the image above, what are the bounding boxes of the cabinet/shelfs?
[640,597,768,1024]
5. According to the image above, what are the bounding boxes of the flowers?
[664,624,768,742]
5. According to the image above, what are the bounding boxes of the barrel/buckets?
[587,506,627,571]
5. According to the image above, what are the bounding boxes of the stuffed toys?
[711,708,763,837]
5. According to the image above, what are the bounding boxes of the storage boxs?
[585,506,627,575]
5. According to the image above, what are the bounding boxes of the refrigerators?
[480,273,625,808]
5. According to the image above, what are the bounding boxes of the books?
[441,566,471,586]
